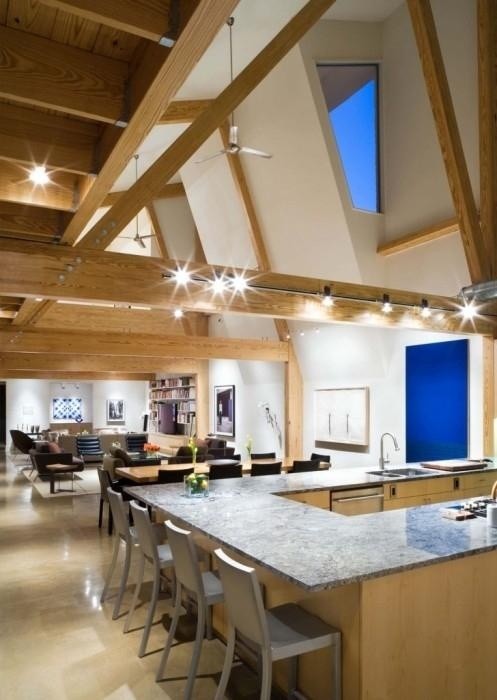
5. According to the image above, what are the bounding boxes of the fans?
[116,151,156,251]
[190,15,274,168]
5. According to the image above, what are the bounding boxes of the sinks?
[368,472,402,478]
[390,468,428,476]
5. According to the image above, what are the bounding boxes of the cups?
[485,504,497,528]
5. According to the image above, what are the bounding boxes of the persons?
[218,400,224,425]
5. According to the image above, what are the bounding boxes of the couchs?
[10,425,97,498]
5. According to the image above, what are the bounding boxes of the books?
[147,376,194,436]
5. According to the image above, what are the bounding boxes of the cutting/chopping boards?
[419,459,487,472]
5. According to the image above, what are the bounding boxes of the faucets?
[378,432,400,469]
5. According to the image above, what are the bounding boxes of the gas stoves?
[460,496,497,519]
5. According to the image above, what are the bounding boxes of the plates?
[204,458,241,468]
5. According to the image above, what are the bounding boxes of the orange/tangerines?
[185,473,207,489]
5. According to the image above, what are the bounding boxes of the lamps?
[314,282,435,320]
[57,381,80,389]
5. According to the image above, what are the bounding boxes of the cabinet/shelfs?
[311,385,370,448]
[149,373,198,435]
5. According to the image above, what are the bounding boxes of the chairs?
[210,549,346,698]
[122,496,218,660]
[96,431,331,535]
[154,517,266,699]
[98,484,172,617]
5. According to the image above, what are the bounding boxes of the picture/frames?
[50,397,84,421]
[104,398,126,424]
[212,383,236,438]
